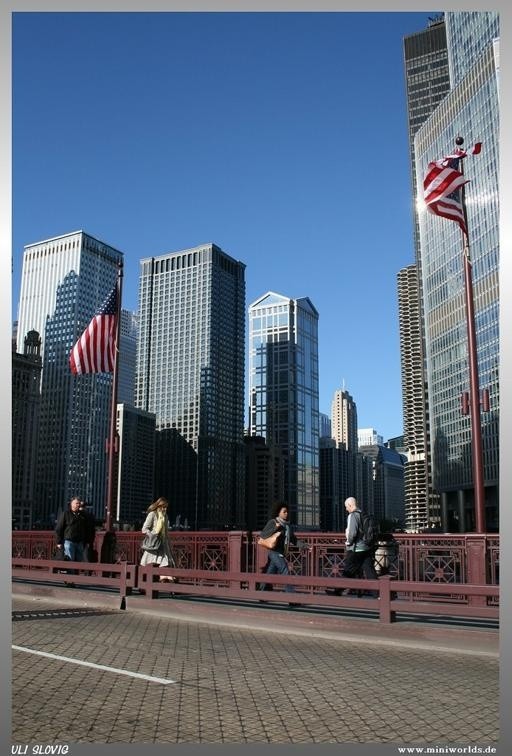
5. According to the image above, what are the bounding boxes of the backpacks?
[352,511,379,546]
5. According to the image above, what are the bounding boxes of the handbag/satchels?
[141,535,161,551]
[258,530,281,549]
[52,549,70,571]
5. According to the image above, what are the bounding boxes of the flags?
[66,279,118,378]
[413,135,485,263]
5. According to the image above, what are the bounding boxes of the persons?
[255,502,311,609]
[74,502,97,577]
[325,496,383,598]
[135,496,180,600]
[53,496,87,588]
